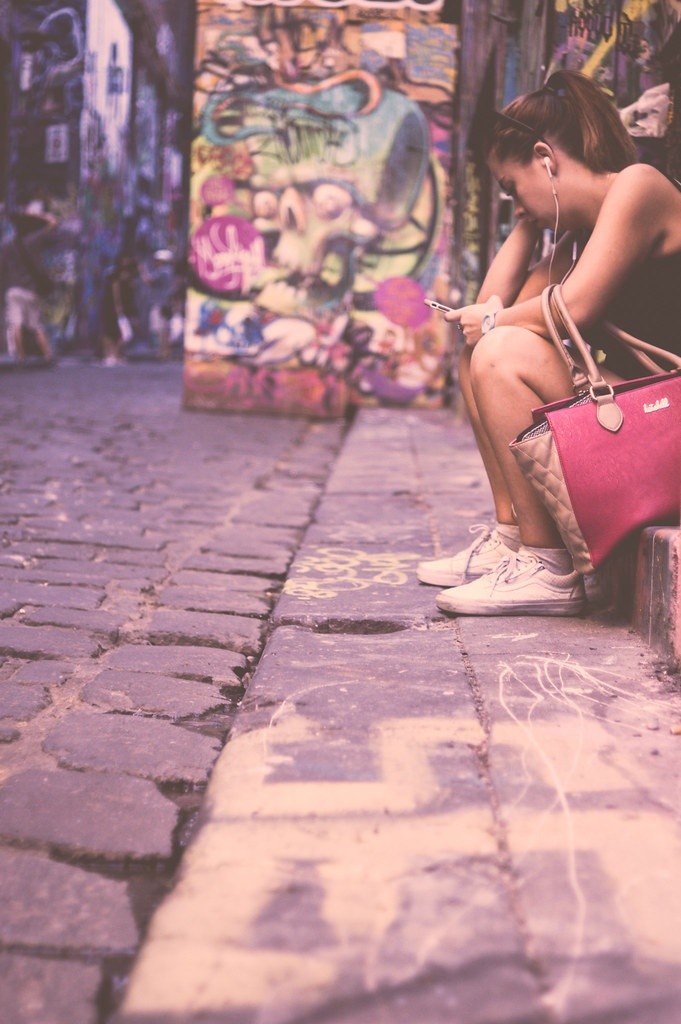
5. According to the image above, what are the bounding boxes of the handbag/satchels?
[509,284,681,575]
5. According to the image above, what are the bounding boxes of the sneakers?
[436,549,586,617]
[417,524,518,587]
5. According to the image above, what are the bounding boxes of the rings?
[456,324,463,334]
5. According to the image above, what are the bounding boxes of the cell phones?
[425,300,455,314]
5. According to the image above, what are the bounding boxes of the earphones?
[544,157,553,180]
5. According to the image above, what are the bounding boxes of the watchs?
[481,307,499,335]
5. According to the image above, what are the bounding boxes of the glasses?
[479,107,548,146]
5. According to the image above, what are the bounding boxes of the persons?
[0,201,177,366]
[417,71,681,617]
